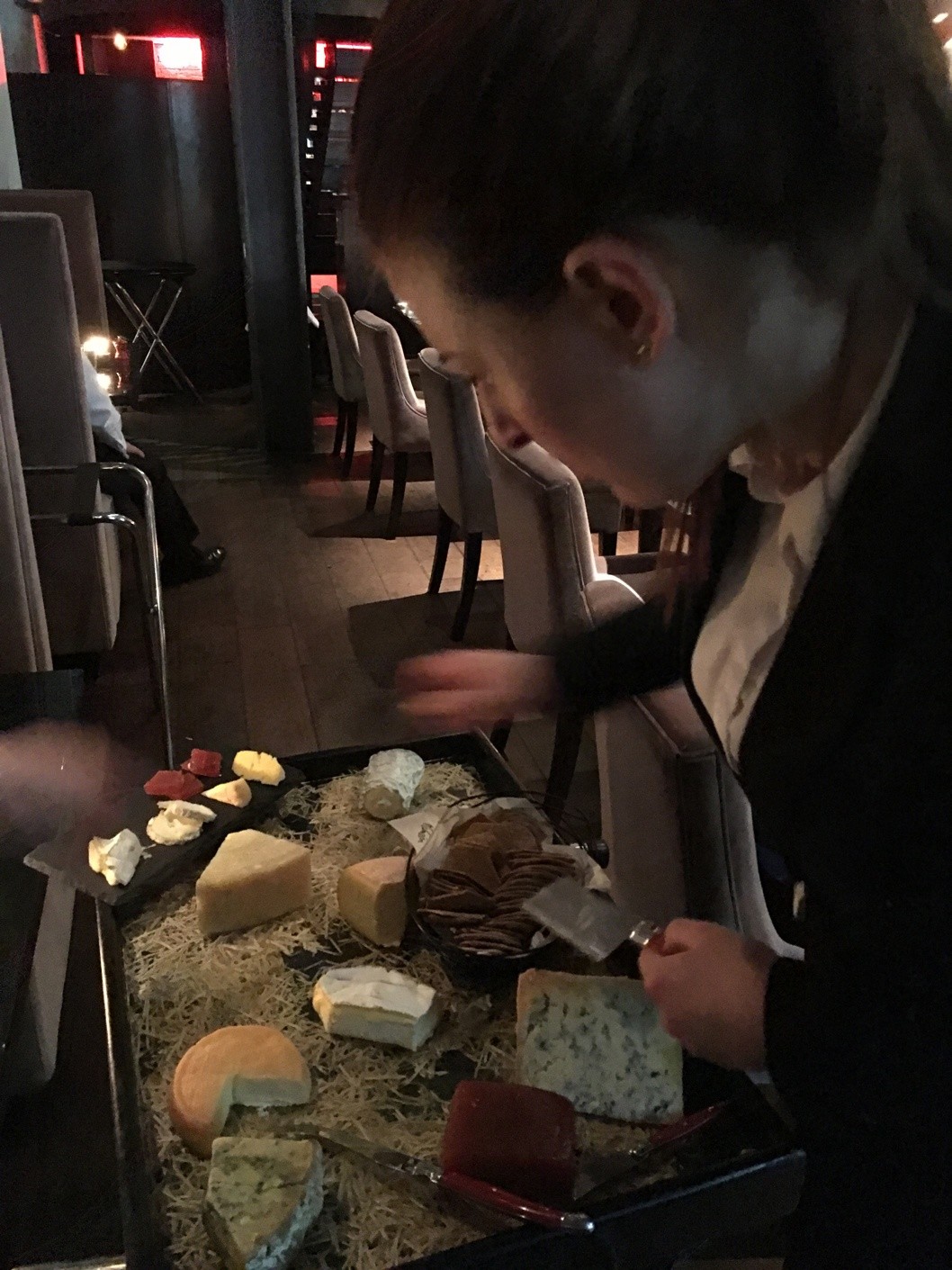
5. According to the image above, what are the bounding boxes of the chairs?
[354,309,430,540]
[417,347,620,643]
[483,432,669,829]
[319,285,422,482]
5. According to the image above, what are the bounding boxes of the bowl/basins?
[405,868,563,972]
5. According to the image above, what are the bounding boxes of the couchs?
[0,190,123,652]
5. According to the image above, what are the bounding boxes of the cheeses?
[87,750,685,1270]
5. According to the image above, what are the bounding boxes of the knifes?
[505,1095,734,1224]
[521,876,672,964]
[290,1124,597,1232]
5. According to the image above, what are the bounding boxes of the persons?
[1,719,155,869]
[347,0,952,1270]
[81,347,226,585]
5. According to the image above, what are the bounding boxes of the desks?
[86,338,136,407]
[101,260,205,404]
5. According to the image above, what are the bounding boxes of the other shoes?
[151,547,228,589]
